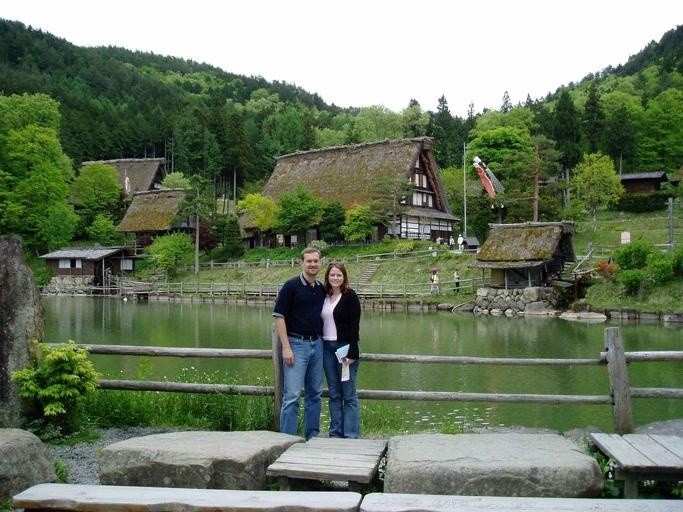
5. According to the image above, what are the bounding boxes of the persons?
[436,234,464,252]
[125,176,131,195]
[453,267,461,293]
[430,268,440,295]
[271,247,361,442]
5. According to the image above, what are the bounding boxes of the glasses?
[329,262,344,267]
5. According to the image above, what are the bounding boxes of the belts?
[287,333,321,340]
